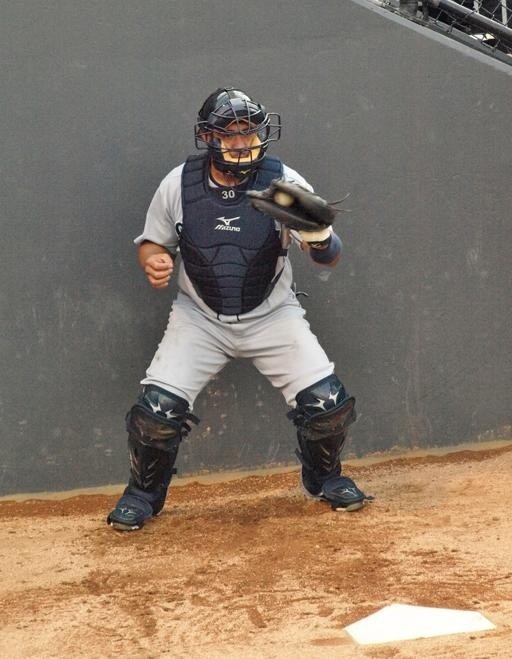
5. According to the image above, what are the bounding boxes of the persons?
[108,86,367,529]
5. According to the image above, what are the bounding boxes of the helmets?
[194,86,283,180]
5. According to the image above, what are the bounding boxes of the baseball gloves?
[245,178,336,230]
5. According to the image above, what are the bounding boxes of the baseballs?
[274,191,294,207]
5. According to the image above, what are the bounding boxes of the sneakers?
[107,496,164,532]
[301,475,365,513]
